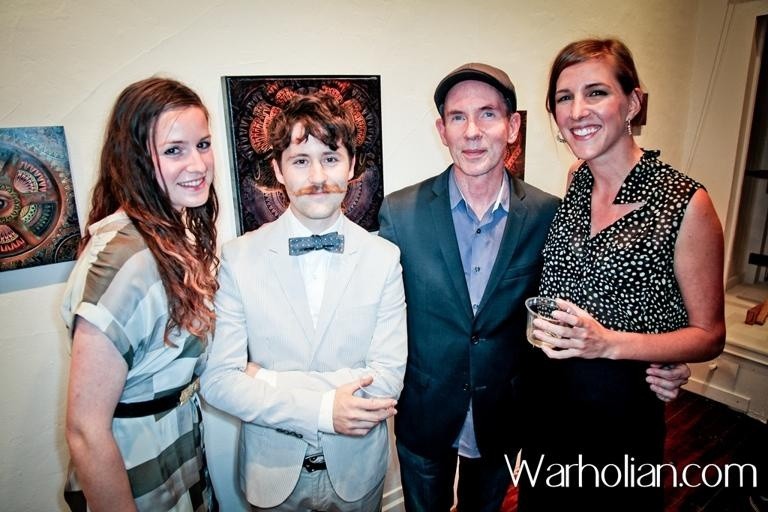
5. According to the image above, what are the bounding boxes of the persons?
[59,38,726,512]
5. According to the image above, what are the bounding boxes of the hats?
[434,63,516,118]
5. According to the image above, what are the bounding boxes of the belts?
[303,459,326,472]
[113,379,193,418]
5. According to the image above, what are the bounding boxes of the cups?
[359,383,398,400]
[525,297,566,349]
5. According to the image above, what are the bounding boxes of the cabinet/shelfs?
[672,280,767,423]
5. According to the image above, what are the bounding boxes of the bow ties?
[289,231,344,256]
